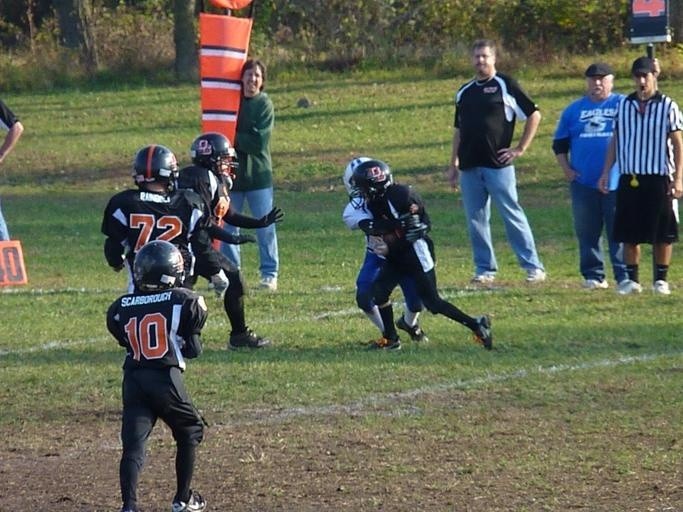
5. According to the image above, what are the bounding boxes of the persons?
[100,141,209,295]
[105,239,211,512]
[178,131,282,348]
[552,64,631,287]
[0,98,26,244]
[597,58,682,294]
[446,39,547,282]
[204,59,281,290]
[343,157,427,341]
[351,160,492,349]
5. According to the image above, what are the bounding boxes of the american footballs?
[384,232,397,246]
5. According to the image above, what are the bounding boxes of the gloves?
[259,207,284,227]
[232,234,255,244]
[209,270,229,299]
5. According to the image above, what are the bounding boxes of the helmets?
[133,145,178,192]
[344,157,392,210]
[192,134,238,185]
[134,241,183,291]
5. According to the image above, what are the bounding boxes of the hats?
[586,63,612,76]
[633,58,654,73]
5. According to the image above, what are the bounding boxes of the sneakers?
[471,272,494,284]
[171,490,207,512]
[259,277,278,290]
[475,315,492,349]
[229,330,271,350]
[525,270,544,282]
[584,279,669,295]
[366,316,429,350]
